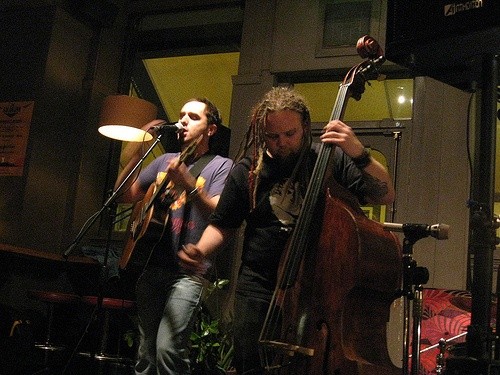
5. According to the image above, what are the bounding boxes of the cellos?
[257,35,405,375]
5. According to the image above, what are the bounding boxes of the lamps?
[97,95,158,238]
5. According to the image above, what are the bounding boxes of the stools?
[28,290,80,375]
[82,295,137,375]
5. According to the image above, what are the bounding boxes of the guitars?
[120,134,205,277]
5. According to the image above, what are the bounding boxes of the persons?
[112,96,236,374]
[178,86,396,375]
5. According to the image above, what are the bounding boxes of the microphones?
[382,222,451,240]
[149,123,183,133]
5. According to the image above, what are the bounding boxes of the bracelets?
[189,186,201,197]
[351,149,371,170]
